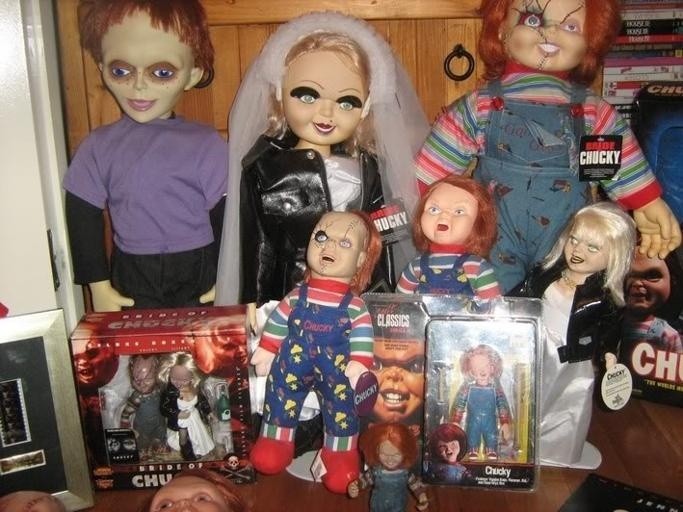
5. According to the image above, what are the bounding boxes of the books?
[599,7,681,131]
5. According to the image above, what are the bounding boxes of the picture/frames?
[0,308,95,512]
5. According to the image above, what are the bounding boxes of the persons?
[408,1,682,302]
[2,305,562,512]
[59,0,231,318]
[222,211,382,489]
[2,172,638,512]
[506,204,641,470]
[214,10,437,334]
[620,239,683,354]
[394,175,502,300]
